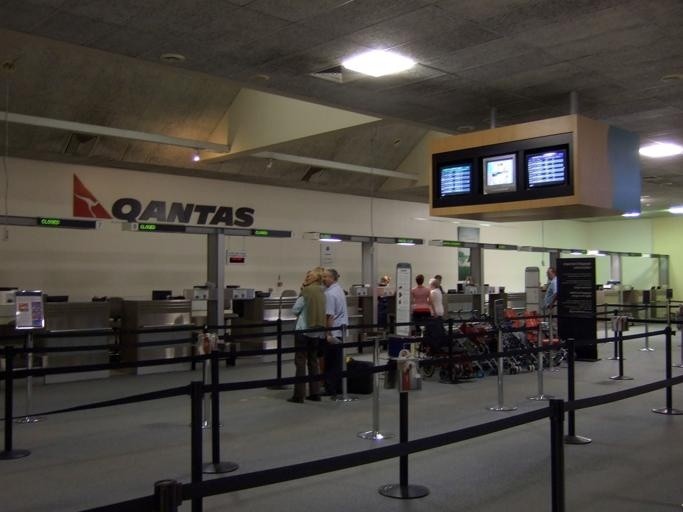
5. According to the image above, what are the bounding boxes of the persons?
[379,275,392,289]
[428,278,445,321]
[320,267,348,398]
[433,274,444,295]
[409,273,437,338]
[544,267,559,331]
[286,269,327,404]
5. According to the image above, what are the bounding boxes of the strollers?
[416,308,568,381]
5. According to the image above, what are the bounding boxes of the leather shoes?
[320,392,336,396]
[288,398,303,403]
[306,396,320,401]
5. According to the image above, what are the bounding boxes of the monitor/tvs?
[439,162,473,199]
[596,284,603,290]
[482,154,517,194]
[448,288,457,293]
[525,149,568,188]
[254,291,271,297]
[152,290,173,300]
[499,287,505,292]
[47,295,69,302]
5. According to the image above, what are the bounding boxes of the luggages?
[346,360,373,395]
